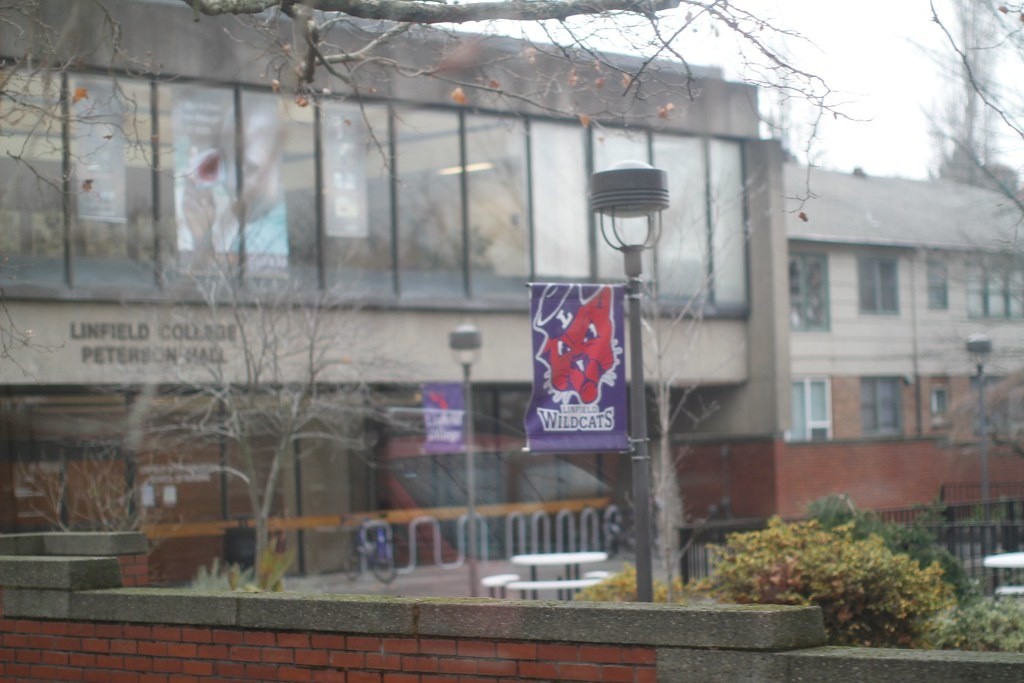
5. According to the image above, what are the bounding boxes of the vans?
[370,427,620,568]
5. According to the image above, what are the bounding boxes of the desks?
[512,552,608,601]
[983,552,1024,587]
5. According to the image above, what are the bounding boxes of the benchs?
[996,587,1024,595]
[480,571,611,600]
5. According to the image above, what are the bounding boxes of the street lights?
[588,158,669,601]
[452,324,484,593]
[967,330,993,557]
